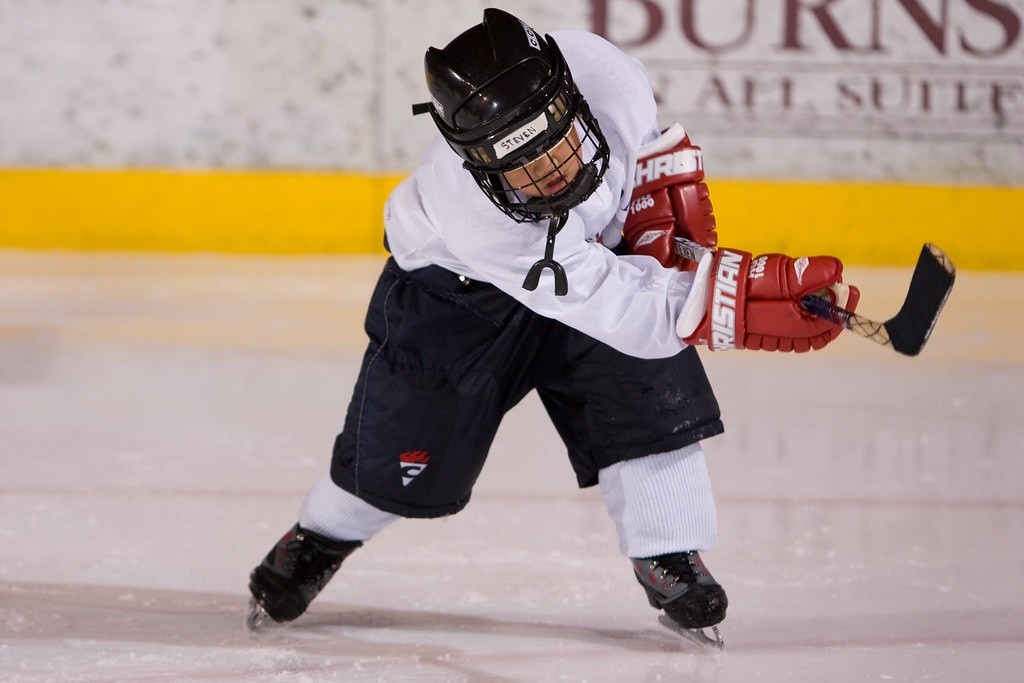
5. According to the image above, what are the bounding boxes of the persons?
[247,7,861,631]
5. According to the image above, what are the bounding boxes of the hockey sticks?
[673,237,955,358]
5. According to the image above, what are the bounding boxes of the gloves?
[622,123,718,271]
[675,247,860,353]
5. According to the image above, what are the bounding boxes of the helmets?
[412,7,611,224]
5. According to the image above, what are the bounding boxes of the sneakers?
[629,550,729,653]
[247,522,362,630]
